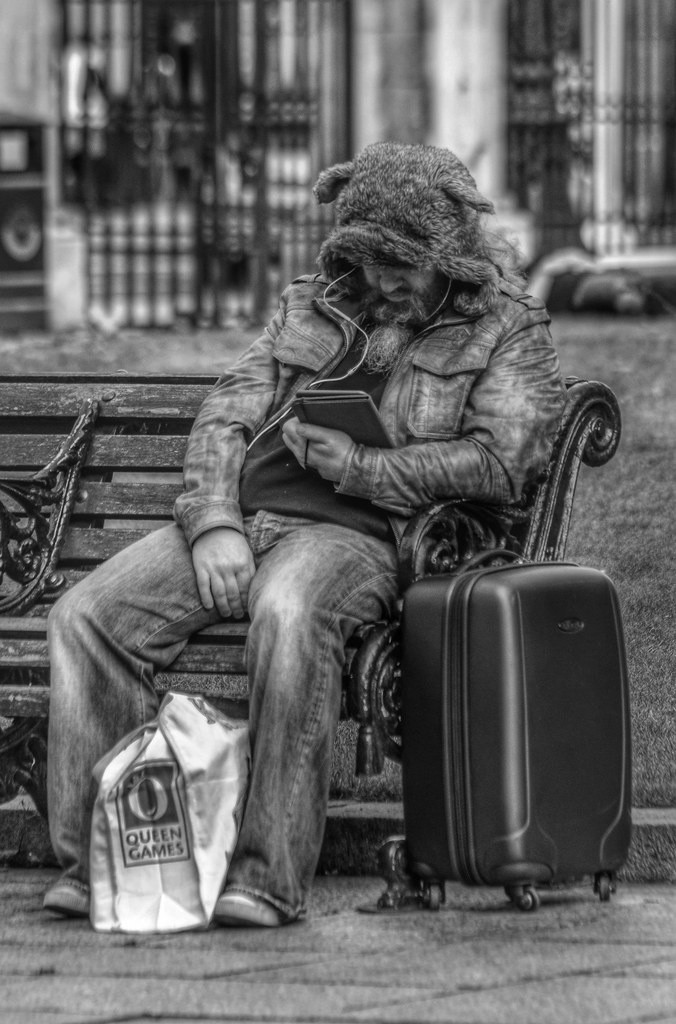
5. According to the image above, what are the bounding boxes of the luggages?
[399,550,633,913]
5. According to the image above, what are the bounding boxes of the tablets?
[291,389,396,452]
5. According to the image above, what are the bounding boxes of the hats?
[313,141,501,318]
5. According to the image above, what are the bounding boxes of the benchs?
[0,372,620,910]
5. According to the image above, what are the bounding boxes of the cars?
[198,97,315,282]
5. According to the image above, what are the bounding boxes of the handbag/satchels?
[89,692,252,934]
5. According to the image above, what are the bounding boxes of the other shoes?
[43,886,90,915]
[214,889,288,927]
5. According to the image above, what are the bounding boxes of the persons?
[45,139,568,929]
